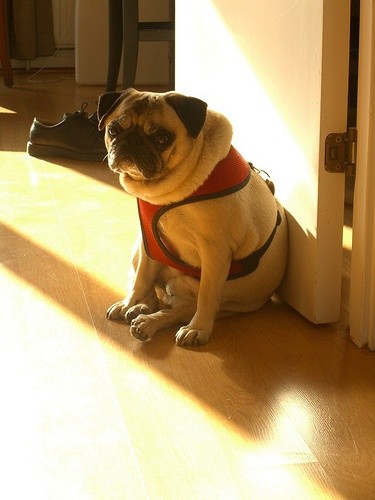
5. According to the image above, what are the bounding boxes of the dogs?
[94,86,291,350]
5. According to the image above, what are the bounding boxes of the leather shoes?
[27,101,108,161]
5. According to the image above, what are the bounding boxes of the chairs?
[100,0,175,94]
